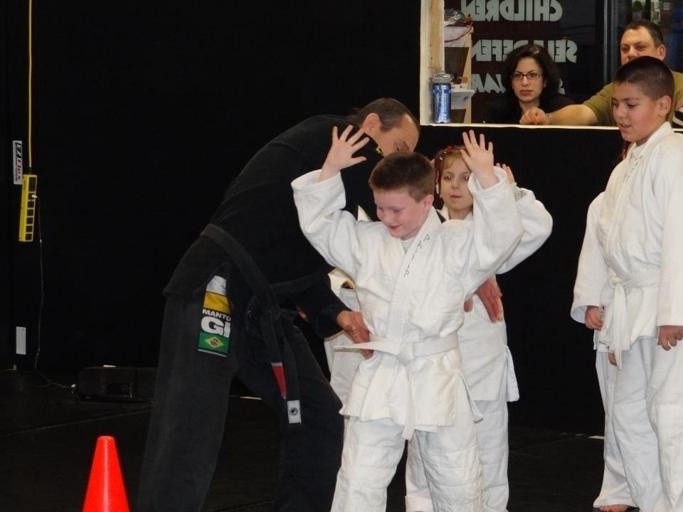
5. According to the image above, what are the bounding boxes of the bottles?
[432,73,452,123]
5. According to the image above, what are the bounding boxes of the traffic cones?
[82,437,128,512]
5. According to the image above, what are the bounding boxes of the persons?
[516,18,681,128]
[401,142,554,511]
[594,57,681,511]
[282,122,522,512]
[484,43,577,122]
[567,136,634,511]
[133,98,504,512]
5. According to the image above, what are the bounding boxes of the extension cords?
[18,174,38,243]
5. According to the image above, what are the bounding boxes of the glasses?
[512,71,543,80]
